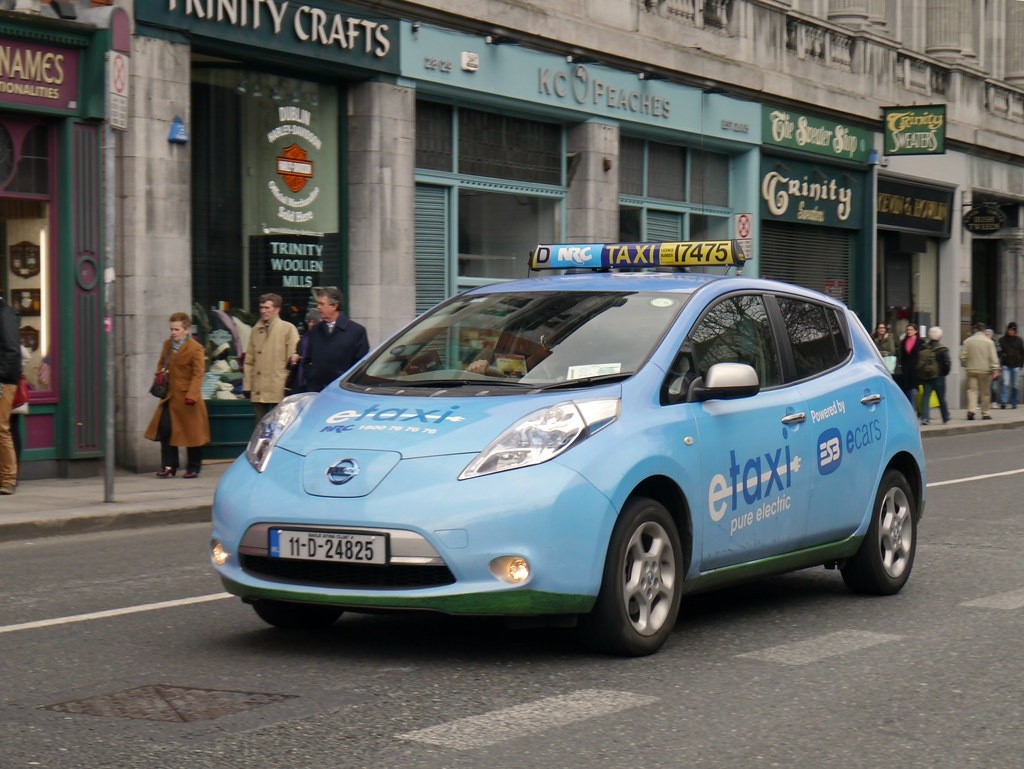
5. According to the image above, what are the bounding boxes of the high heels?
[156,467,176,478]
[182,471,197,478]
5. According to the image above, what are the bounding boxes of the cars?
[211,239,926,659]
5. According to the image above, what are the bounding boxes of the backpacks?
[917,344,945,380]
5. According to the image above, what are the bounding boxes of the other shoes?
[1012,407,1016,409]
[982,416,991,420]
[944,418,952,425]
[1000,405,1005,409]
[967,411,974,420]
[920,419,929,425]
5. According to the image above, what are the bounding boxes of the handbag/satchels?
[149,371,168,399]
[915,384,940,416]
[12,376,29,409]
[882,355,897,374]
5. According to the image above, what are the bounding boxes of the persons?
[898,323,925,415]
[284,286,369,402]
[999,322,1024,409]
[870,322,895,376]
[143,313,211,478]
[983,329,1002,409]
[242,293,301,428]
[961,322,1000,420]
[467,321,567,379]
[917,326,951,425]
[0,295,22,494]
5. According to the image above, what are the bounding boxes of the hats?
[971,322,984,329]
[928,327,943,340]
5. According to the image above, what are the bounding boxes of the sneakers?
[1,482,16,496]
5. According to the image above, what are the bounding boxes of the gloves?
[243,390,251,398]
